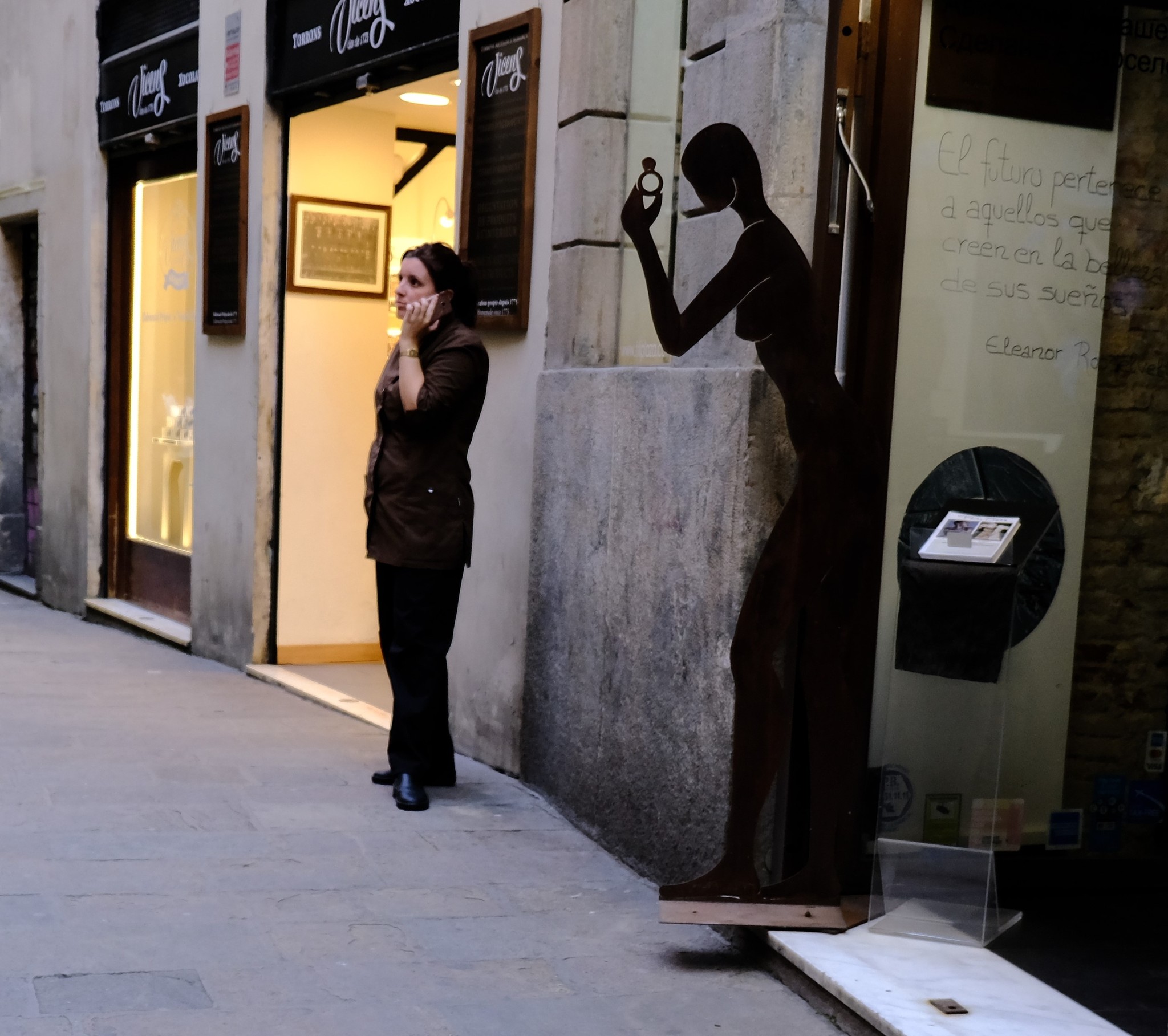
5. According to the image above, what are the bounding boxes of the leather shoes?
[372,771,457,787]
[393,773,429,812]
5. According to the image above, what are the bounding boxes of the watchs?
[398,348,419,358]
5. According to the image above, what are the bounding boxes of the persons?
[365,243,489,812]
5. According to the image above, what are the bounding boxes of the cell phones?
[423,293,453,325]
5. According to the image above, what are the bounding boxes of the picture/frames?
[286,195,392,299]
